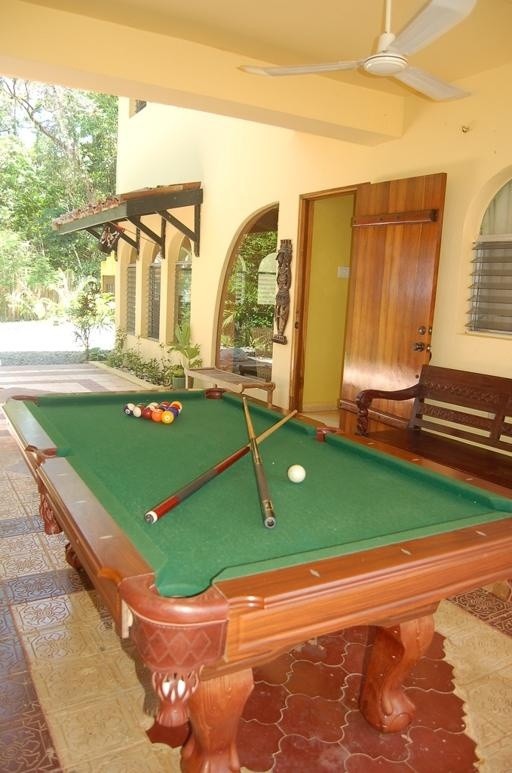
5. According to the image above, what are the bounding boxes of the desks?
[1,387,512,773]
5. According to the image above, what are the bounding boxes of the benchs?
[185,366,276,404]
[356,364,512,488]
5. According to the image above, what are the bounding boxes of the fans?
[236,1,476,102]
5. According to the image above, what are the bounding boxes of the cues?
[145,408,299,523]
[241,393,279,527]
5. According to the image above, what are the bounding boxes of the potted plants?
[172,369,185,389]
